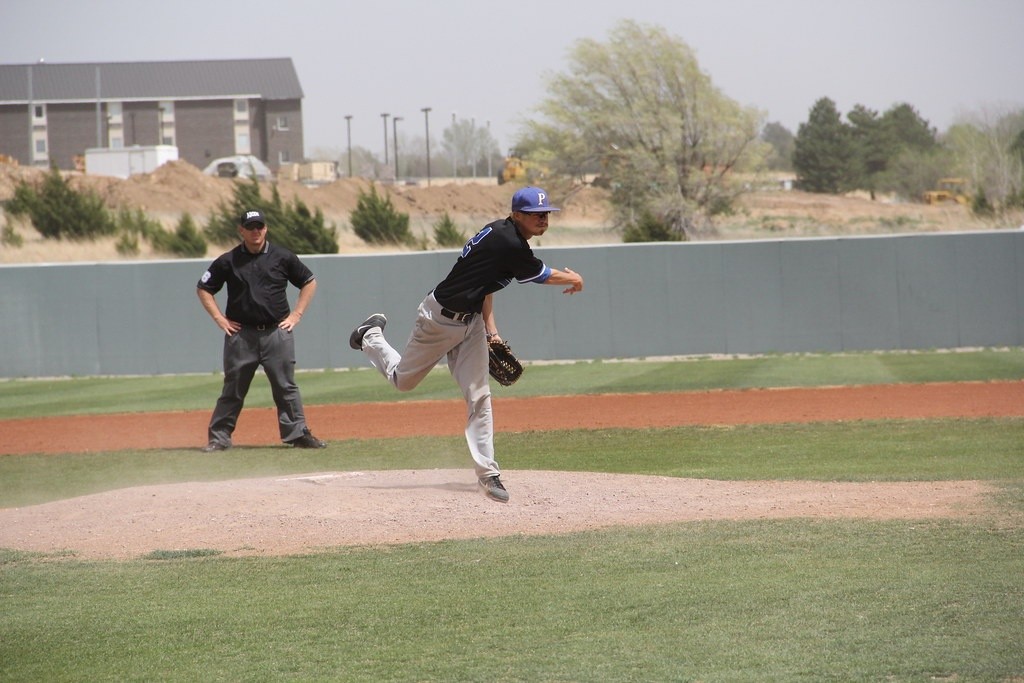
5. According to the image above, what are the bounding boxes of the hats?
[240,210,266,226]
[511,186,561,212]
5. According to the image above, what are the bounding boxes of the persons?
[349,186,582,503]
[196,209,326,453]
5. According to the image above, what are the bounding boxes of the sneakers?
[477,474,509,504]
[350,313,387,351]
[284,426,327,447]
[205,442,227,452]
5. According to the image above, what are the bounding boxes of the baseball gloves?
[488,340,524,386]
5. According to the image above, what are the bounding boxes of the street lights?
[345,116,352,178]
[381,114,390,164]
[422,108,432,187]
[394,118,402,177]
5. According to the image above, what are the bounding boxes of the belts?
[251,323,276,331]
[427,288,473,323]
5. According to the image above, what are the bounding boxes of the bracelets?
[487,331,498,338]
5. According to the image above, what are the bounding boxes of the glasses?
[242,222,265,230]
